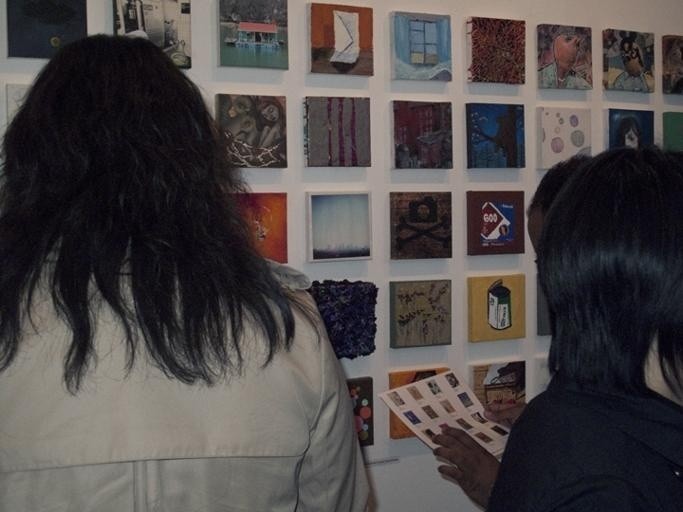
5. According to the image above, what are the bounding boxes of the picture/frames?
[305,192,374,261]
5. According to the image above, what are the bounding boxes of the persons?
[254,96,286,149]
[431,145,682,510]
[0,31,376,512]
[431,152,596,512]
